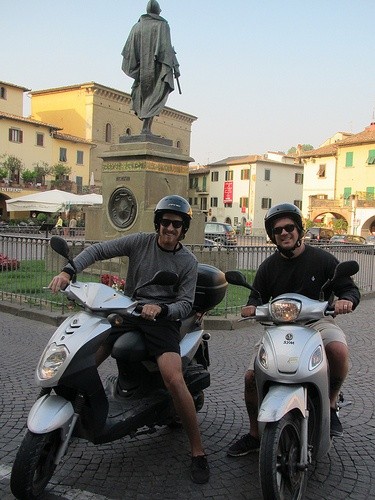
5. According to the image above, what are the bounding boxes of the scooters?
[9,236,228,500]
[225,260,360,500]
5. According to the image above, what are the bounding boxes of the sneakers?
[190,447,209,484]
[227,432,260,456]
[330,407,343,436]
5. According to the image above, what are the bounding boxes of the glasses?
[159,219,184,229]
[272,224,297,235]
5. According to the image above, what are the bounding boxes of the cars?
[205,222,237,245]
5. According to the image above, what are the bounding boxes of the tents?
[5,189,77,213]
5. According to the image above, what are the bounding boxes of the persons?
[228,204,360,457]
[56,216,63,235]
[49,194,210,484]
[69,218,77,236]
[121,0,180,135]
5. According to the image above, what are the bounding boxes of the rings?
[344,305,347,306]
[151,312,153,314]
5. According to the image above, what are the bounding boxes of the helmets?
[154,195,192,220]
[264,202,303,244]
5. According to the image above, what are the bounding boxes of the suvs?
[304,227,375,248]
